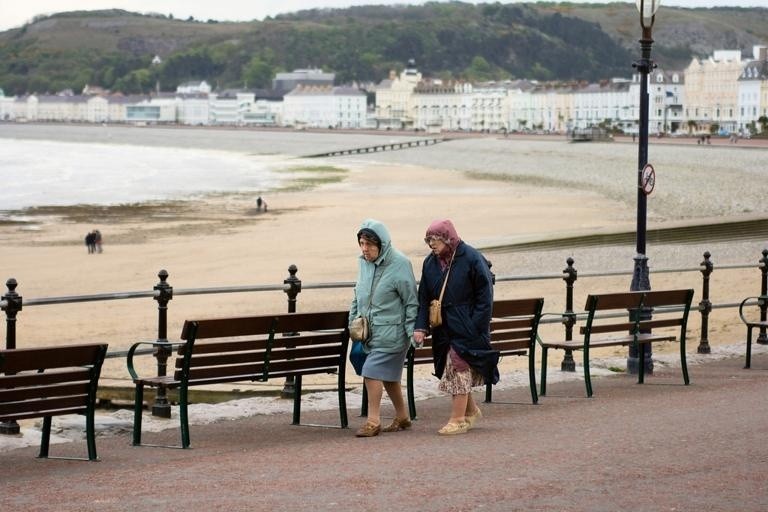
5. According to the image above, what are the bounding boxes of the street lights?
[626,0,661,375]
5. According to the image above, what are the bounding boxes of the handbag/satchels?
[349,318,369,342]
[429,301,443,327]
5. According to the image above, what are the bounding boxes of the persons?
[84,229,103,255]
[256,197,263,212]
[263,202,268,212]
[347,218,424,437]
[412,219,502,436]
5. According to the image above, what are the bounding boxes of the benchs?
[124,308,355,451]
[538,284,696,400]
[736,291,767,371]
[360,294,545,424]
[1,341,112,465]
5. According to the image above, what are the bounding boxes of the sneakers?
[356,420,381,437]
[438,410,482,434]
[383,416,412,431]
